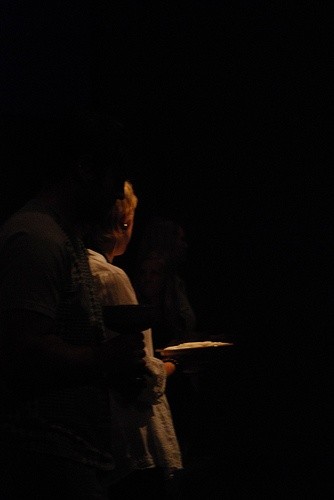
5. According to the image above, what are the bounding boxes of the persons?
[73,175,195,500]
[0,118,146,500]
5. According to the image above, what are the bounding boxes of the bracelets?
[162,357,179,366]
